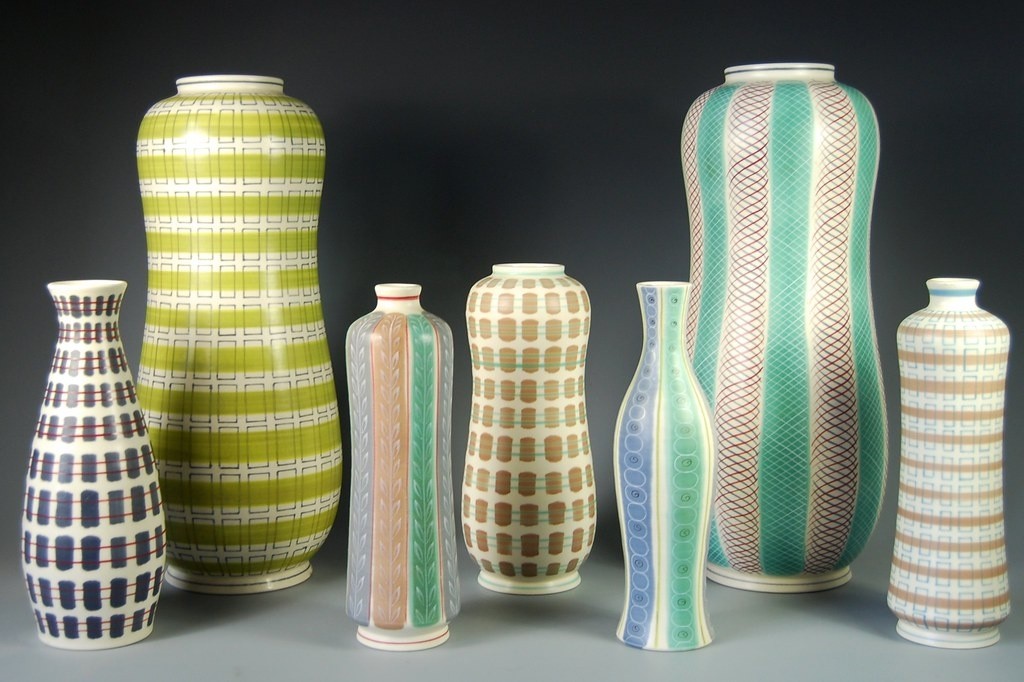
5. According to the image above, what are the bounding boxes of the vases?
[458,263,598,595]
[341,283,463,652]
[19,279,166,653]
[613,281,718,652]
[129,72,345,597]
[684,62,890,596]
[885,276,1012,651]
